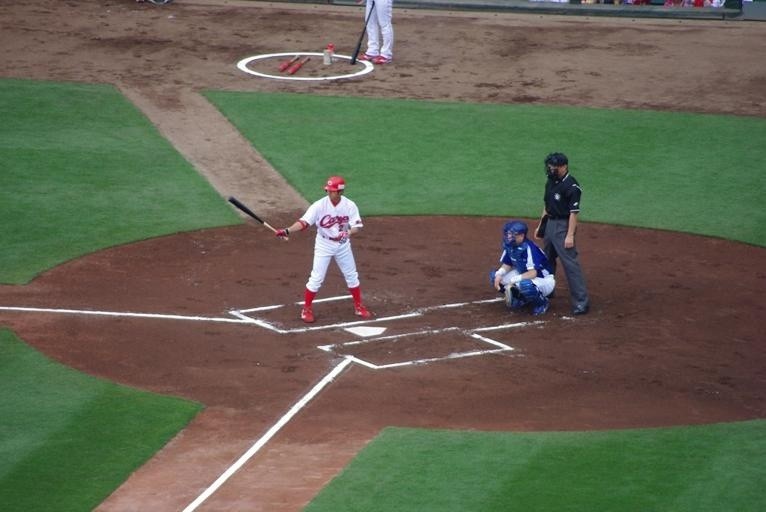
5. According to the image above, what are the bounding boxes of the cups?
[323,50,331,66]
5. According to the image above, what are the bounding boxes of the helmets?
[323,176,344,192]
[502,221,527,250]
[545,152,566,182]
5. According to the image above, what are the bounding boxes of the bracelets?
[495,268,506,276]
[511,275,523,283]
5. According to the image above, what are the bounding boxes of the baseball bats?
[279,55,300,71]
[351,1,374,66]
[289,57,310,74]
[229,195,290,242]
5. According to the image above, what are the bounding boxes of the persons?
[489,219,555,316]
[359,1,394,66]
[273,175,376,321]
[534,152,590,312]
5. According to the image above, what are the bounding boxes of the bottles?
[327,43,334,61]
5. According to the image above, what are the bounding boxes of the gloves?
[336,232,349,243]
[272,229,288,239]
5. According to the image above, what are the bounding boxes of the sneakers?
[300,306,313,323]
[533,297,550,316]
[574,305,586,315]
[354,307,370,317]
[358,54,391,64]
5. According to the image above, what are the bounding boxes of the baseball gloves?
[505,283,519,308]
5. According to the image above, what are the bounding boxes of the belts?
[323,236,336,240]
